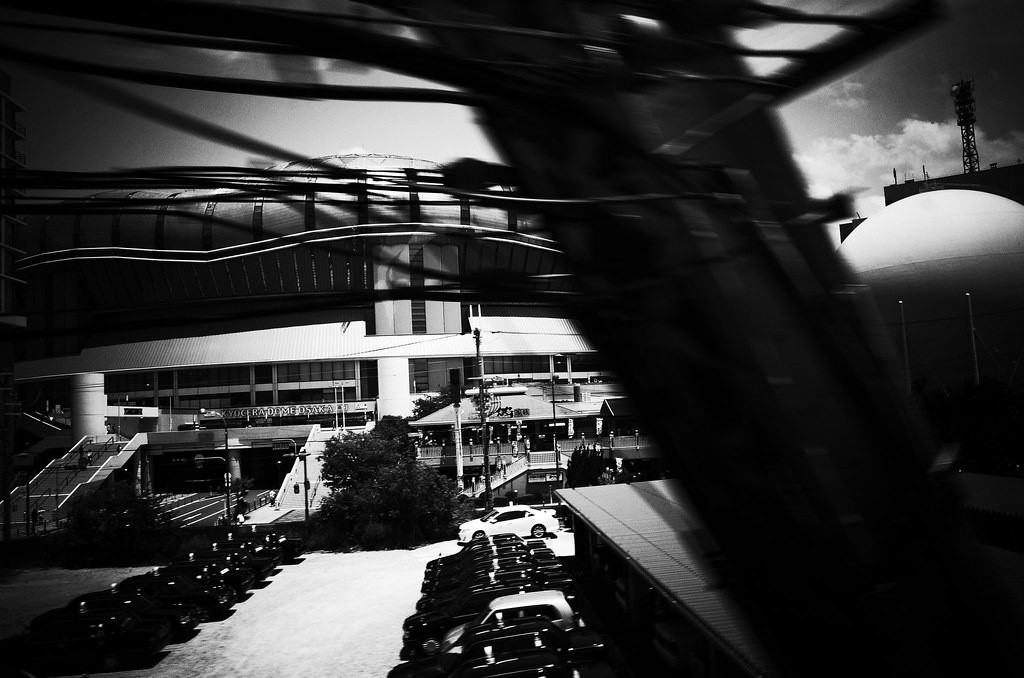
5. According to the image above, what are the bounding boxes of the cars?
[401,532,607,678]
[459,504,559,543]
[25,531,312,668]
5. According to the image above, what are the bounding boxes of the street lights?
[552,374,560,491]
[197,409,230,525]
[284,454,309,526]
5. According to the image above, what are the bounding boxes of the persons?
[30,507,44,526]
[79,444,92,461]
[294,478,310,494]
[269,488,276,507]
[117,445,122,454]
[219,513,228,525]
[422,427,453,446]
[236,512,245,525]
[364,417,374,435]
